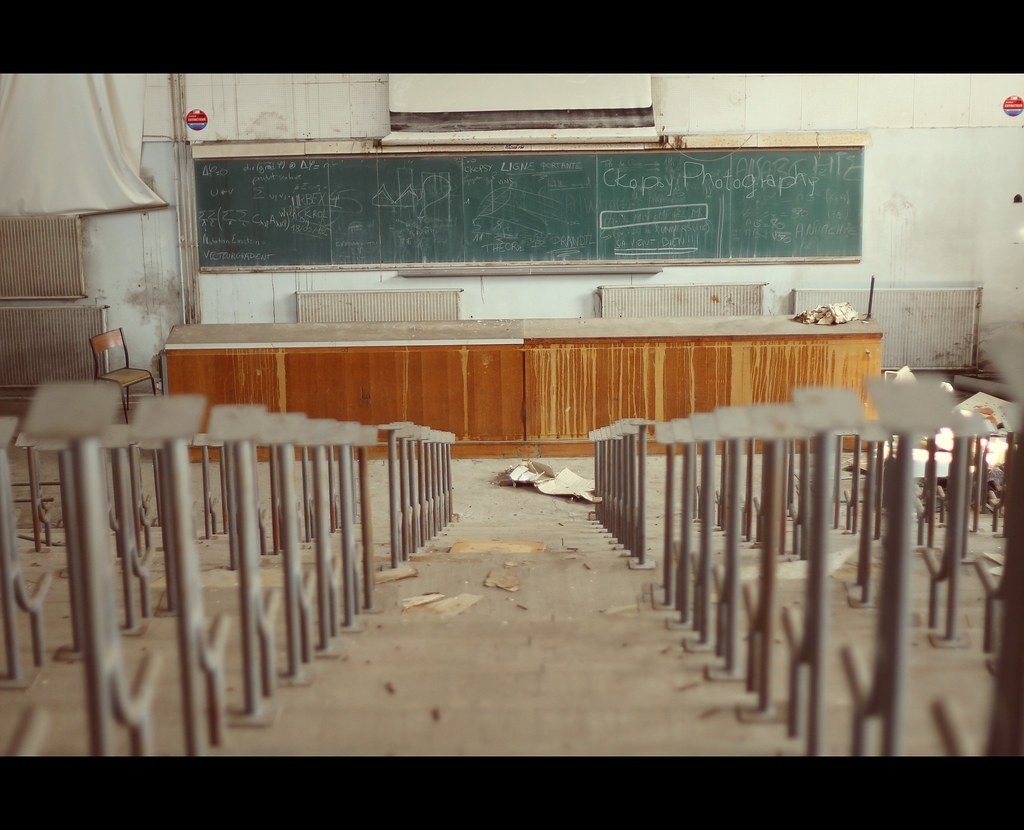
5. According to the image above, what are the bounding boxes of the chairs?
[90,327,158,429]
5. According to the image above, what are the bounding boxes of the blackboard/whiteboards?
[187,131,868,278]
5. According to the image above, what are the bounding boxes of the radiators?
[1,299,113,390]
[0,214,89,302]
[595,282,768,319]
[294,288,466,323]
[790,285,984,374]
